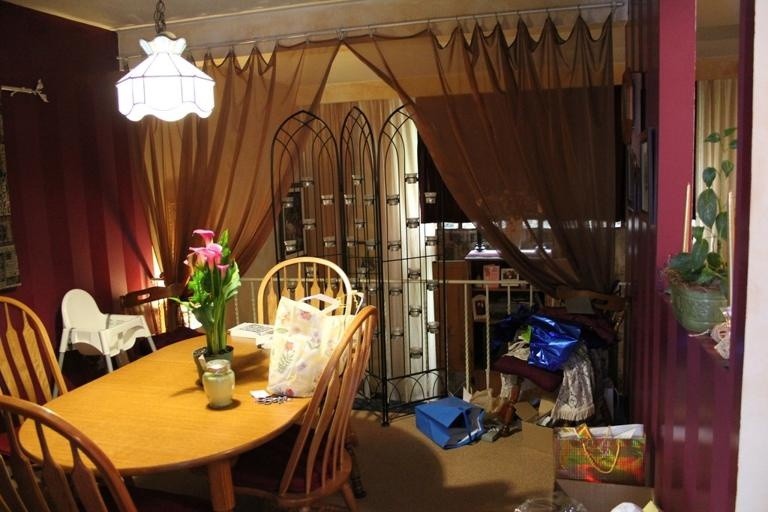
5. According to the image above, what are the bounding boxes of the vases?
[193,346,233,384]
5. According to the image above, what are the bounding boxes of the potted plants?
[668,126,737,331]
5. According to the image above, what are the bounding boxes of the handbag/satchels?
[552,423,648,484]
[267,291,367,398]
[415,396,485,449]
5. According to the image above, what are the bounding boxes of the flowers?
[167,225,242,352]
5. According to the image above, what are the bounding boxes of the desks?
[18,324,355,510]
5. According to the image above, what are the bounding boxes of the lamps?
[115,0,216,123]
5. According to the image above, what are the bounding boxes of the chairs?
[52,290,158,399]
[491,284,626,437]
[2,395,137,510]
[119,285,202,366]
[258,256,352,331]
[230,305,376,509]
[1,296,69,465]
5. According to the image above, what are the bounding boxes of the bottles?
[202,360,237,408]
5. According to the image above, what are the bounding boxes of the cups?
[280,173,440,358]
